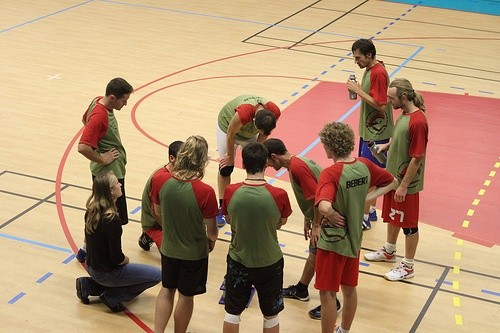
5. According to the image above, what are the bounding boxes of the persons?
[154,135,219,333]
[214,94,281,226]
[364,78,428,281]
[346,39,395,230]
[314,122,399,333]
[76,170,161,313]
[263,138,343,320]
[76,77,133,262]
[219,141,292,333]
[138,141,183,255]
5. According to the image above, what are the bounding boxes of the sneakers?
[369,210,377,221]
[216,208,225,226]
[364,246,396,262]
[283,285,310,301]
[98,293,125,313]
[137,232,155,251]
[309,299,341,320]
[334,325,342,333]
[76,248,87,262]
[76,277,90,304]
[384,261,414,281]
[362,219,371,231]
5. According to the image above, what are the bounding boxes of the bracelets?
[330,209,335,216]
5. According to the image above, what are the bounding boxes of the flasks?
[348,75,357,99]
